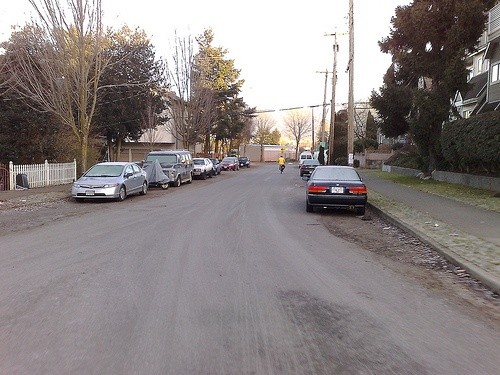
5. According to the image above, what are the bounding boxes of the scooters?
[135,159,170,190]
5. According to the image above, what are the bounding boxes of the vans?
[298,151,314,168]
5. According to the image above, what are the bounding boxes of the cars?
[209,153,251,175]
[71,162,149,202]
[302,165,368,215]
[298,159,323,177]
[193,157,215,180]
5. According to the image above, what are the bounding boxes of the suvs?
[145,149,194,187]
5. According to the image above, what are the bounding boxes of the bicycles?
[278,162,286,174]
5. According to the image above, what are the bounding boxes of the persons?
[278,156,286,171]
